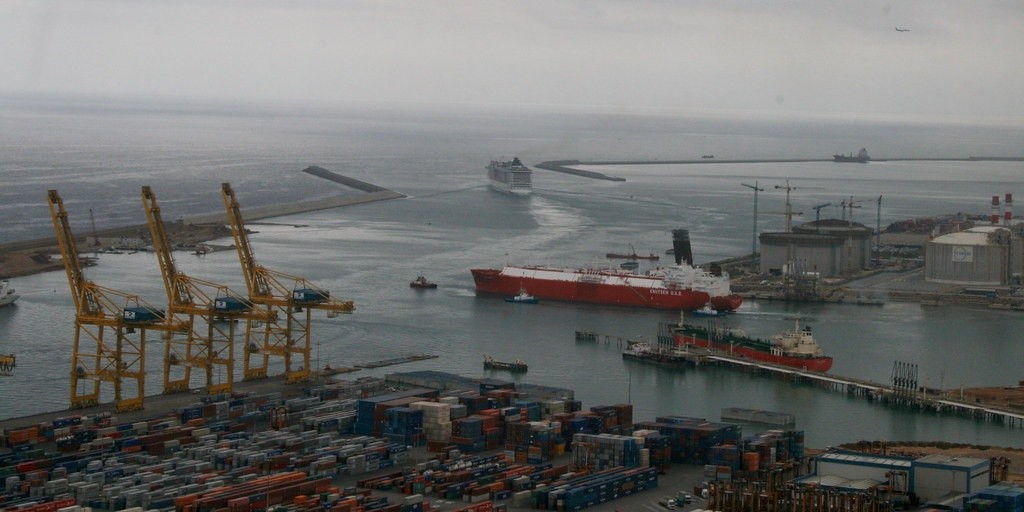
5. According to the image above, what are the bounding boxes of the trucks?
[694,485,710,499]
[659,499,676,510]
[680,490,692,504]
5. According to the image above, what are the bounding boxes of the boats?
[833,152,870,163]
[469,255,743,313]
[691,296,726,318]
[483,355,529,372]
[487,160,535,197]
[410,280,437,289]
[503,278,539,305]
[667,309,833,374]
[0,279,21,307]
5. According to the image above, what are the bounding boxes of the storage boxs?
[0,371,804,512]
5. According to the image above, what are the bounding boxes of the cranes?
[740,179,764,274]
[775,179,797,287]
[46,189,193,416]
[140,185,279,396]
[219,182,357,385]
